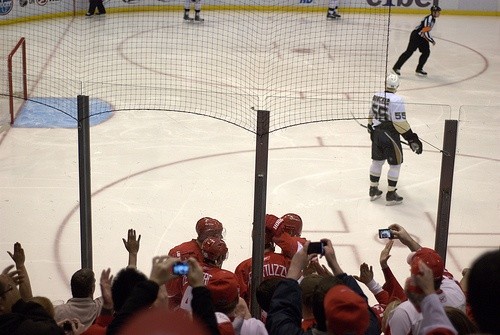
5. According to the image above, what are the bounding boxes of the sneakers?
[96,12,107,16]
[194,16,205,23]
[184,15,193,21]
[385,189,403,206]
[85,13,94,18]
[370,186,383,202]
[392,68,401,76]
[416,67,427,76]
[327,13,336,20]
[334,13,341,19]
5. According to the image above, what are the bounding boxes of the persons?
[0,213,500,335]
[326,0,341,18]
[367,74,423,203]
[184,0,204,21]
[85,0,109,16]
[393,5,441,76]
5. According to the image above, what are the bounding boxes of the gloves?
[408,133,422,154]
[265,214,286,237]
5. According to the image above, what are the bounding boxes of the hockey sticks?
[351,111,410,146]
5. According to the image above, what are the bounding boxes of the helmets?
[282,213,302,235]
[201,237,228,257]
[196,217,223,238]
[431,5,441,12]
[386,74,398,89]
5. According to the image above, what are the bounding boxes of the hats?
[408,247,445,278]
[207,271,238,305]
[323,286,370,335]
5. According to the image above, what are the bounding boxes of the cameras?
[307,242,325,254]
[379,229,394,238]
[172,263,190,276]
